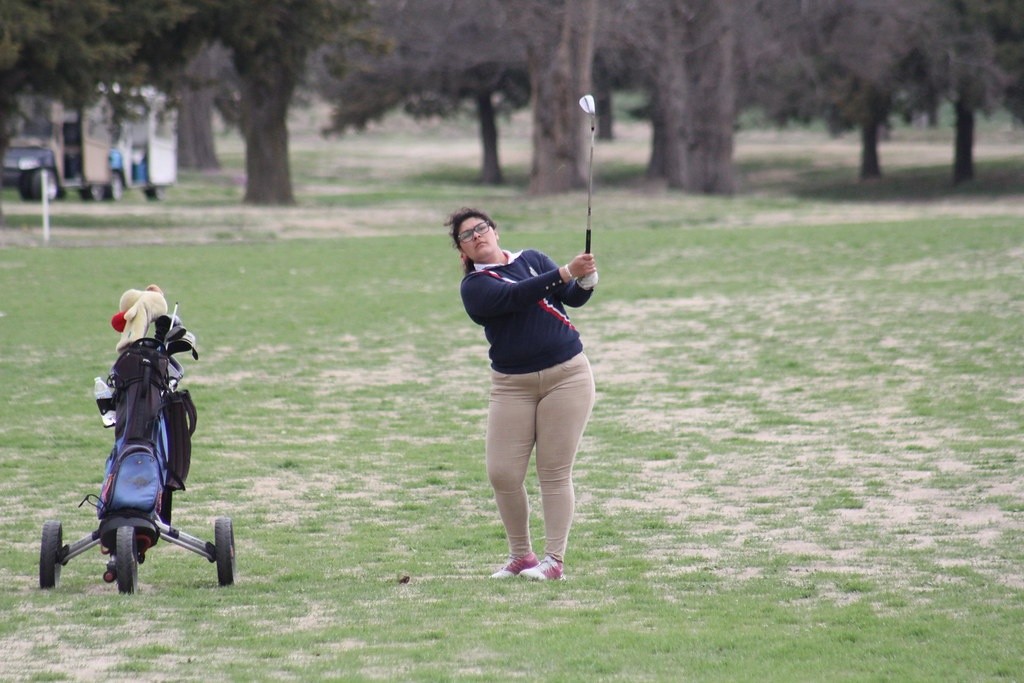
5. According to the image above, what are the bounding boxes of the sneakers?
[490,551,539,583]
[519,556,563,582]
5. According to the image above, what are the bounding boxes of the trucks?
[80,79,180,202]
[0,78,113,202]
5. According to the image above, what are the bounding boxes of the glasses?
[457,220,489,243]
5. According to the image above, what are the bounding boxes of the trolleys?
[39,314,234,588]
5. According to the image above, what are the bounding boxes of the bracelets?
[565,263,576,282]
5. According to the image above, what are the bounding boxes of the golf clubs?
[155,301,199,360]
[579,95,596,254]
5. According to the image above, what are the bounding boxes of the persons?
[443,205,599,581]
